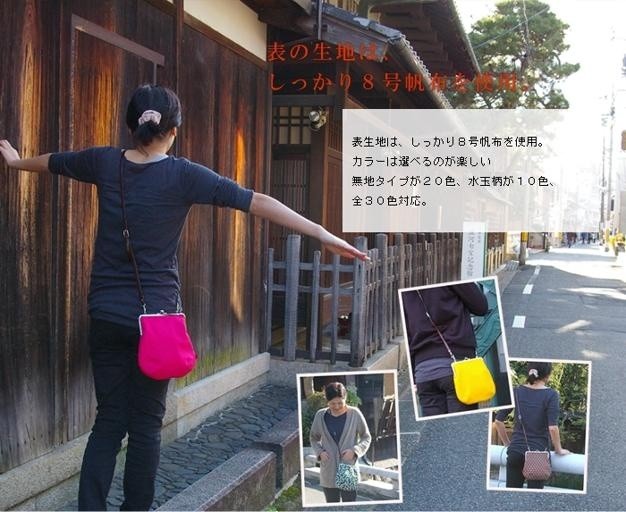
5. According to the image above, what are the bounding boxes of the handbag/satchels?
[136,310,197,381]
[334,464,358,492]
[521,451,551,481]
[450,357,496,405]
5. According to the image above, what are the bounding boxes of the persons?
[0,85,373,512]
[495,363,569,490]
[308,382,372,502]
[402,282,487,417]
[566,232,596,249]
